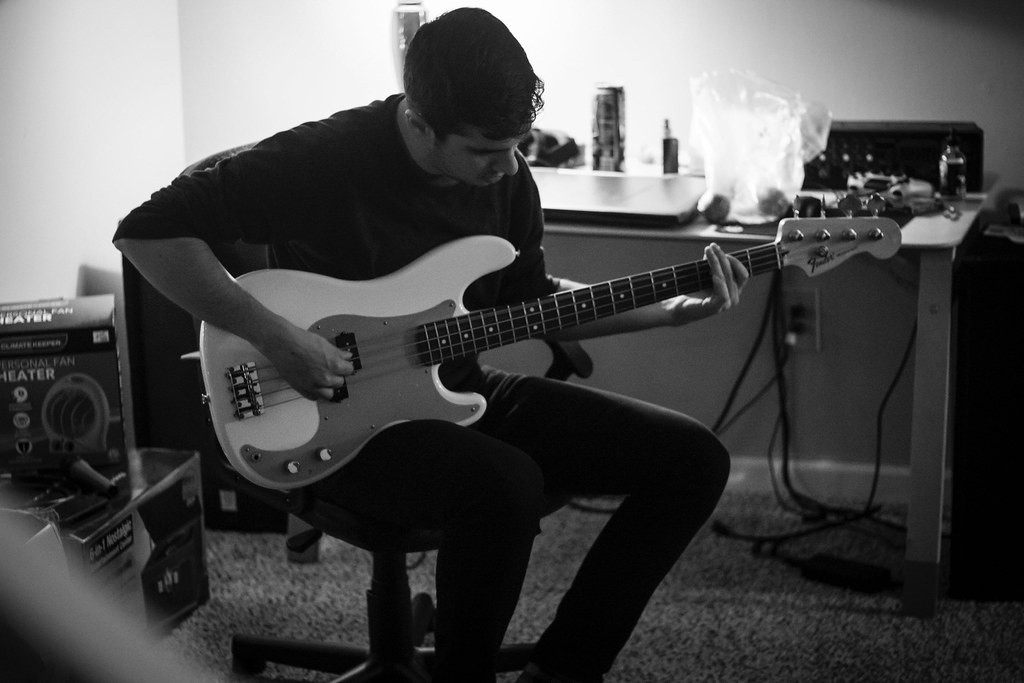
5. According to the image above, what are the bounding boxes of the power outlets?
[780,283,821,354]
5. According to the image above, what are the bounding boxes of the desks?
[542,191,987,567]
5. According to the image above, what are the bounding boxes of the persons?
[112,8,752,683]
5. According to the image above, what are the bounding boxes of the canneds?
[592,85,625,171]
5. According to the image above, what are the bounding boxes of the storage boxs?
[0,445,215,640]
[0,293,129,474]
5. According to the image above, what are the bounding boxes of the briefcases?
[802,119,986,193]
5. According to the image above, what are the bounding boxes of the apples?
[755,186,789,218]
[698,188,731,223]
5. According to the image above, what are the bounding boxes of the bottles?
[937,127,967,211]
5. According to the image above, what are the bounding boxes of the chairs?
[179,144,536,683]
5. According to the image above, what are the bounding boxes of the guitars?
[197,188,904,492]
